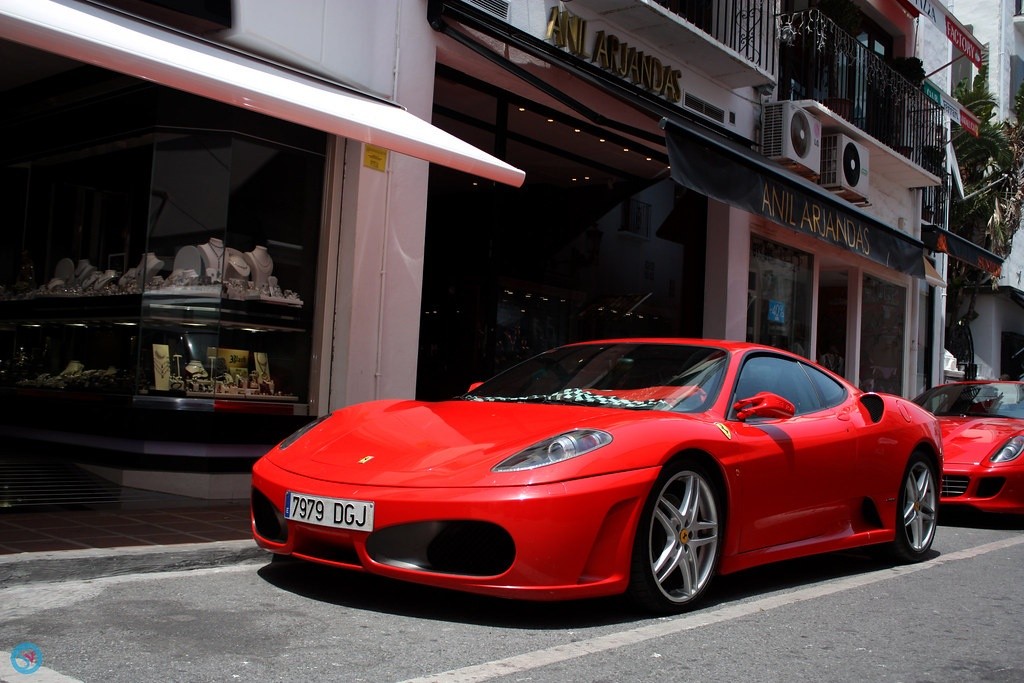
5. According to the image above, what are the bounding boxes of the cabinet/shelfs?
[0,125,326,444]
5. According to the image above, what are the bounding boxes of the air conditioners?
[816,133,869,206]
[761,101,822,178]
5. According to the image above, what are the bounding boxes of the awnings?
[432,0,926,279]
[0,0,525,188]
[924,255,945,288]
[1007,291,1024,308]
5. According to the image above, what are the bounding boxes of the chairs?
[625,361,664,391]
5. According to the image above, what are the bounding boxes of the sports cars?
[247,337,947,614]
[906,377,1024,515]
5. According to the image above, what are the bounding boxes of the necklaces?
[189,363,203,369]
[227,256,248,269]
[75,264,90,277]
[98,276,111,284]
[252,252,272,274]
[138,257,157,275]
[208,241,224,269]
[85,274,99,284]
[257,353,267,373]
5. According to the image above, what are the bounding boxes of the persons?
[860,352,876,391]
[1001,371,1013,380]
[820,346,845,376]
